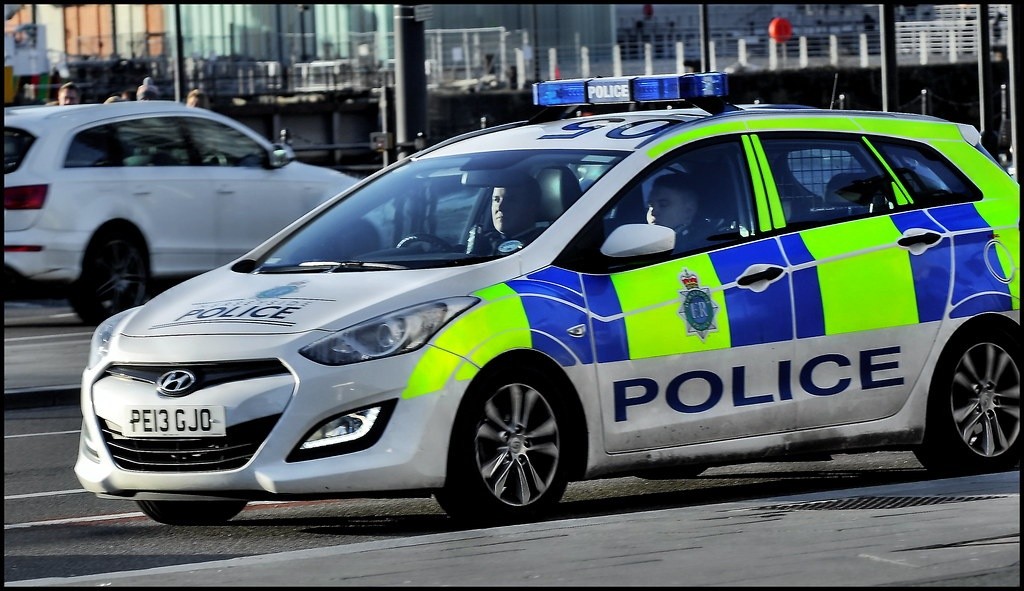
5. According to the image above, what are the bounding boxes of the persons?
[186,89,204,106]
[49,72,61,100]
[647,174,697,231]
[481,177,552,255]
[136,77,159,100]
[58,82,80,105]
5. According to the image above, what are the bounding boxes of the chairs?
[531,165,581,221]
[689,150,745,231]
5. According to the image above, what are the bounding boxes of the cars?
[74,73,1022,534]
[3,100,412,327]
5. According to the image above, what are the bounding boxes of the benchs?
[825,172,866,205]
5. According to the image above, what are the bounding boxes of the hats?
[136,77,159,100]
